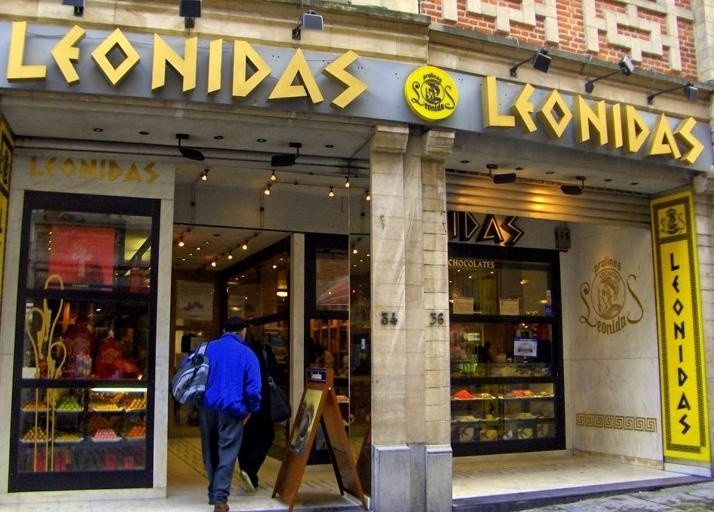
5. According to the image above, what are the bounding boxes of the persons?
[237,322,286,494]
[58,312,97,378]
[474,339,496,362]
[94,317,144,382]
[177,315,263,511]
[526,323,551,364]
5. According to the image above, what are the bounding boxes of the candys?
[20,391,147,442]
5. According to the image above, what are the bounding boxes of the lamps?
[179,0,202,28]
[62,1,85,16]
[176,133,205,161]
[271,141,303,166]
[647,79,699,105]
[486,163,516,184]
[292,9,324,39]
[561,175,586,196]
[585,55,635,93]
[509,48,553,77]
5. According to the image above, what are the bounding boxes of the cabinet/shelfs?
[449,308,558,446]
[20,299,155,477]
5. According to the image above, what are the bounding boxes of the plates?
[17,407,146,443]
[450,394,554,424]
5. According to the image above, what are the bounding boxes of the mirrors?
[350,142,372,493]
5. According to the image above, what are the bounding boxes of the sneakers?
[214,499,229,511]
[238,467,260,494]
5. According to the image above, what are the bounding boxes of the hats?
[223,316,250,331]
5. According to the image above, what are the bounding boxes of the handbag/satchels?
[172,340,211,407]
[261,342,291,422]
